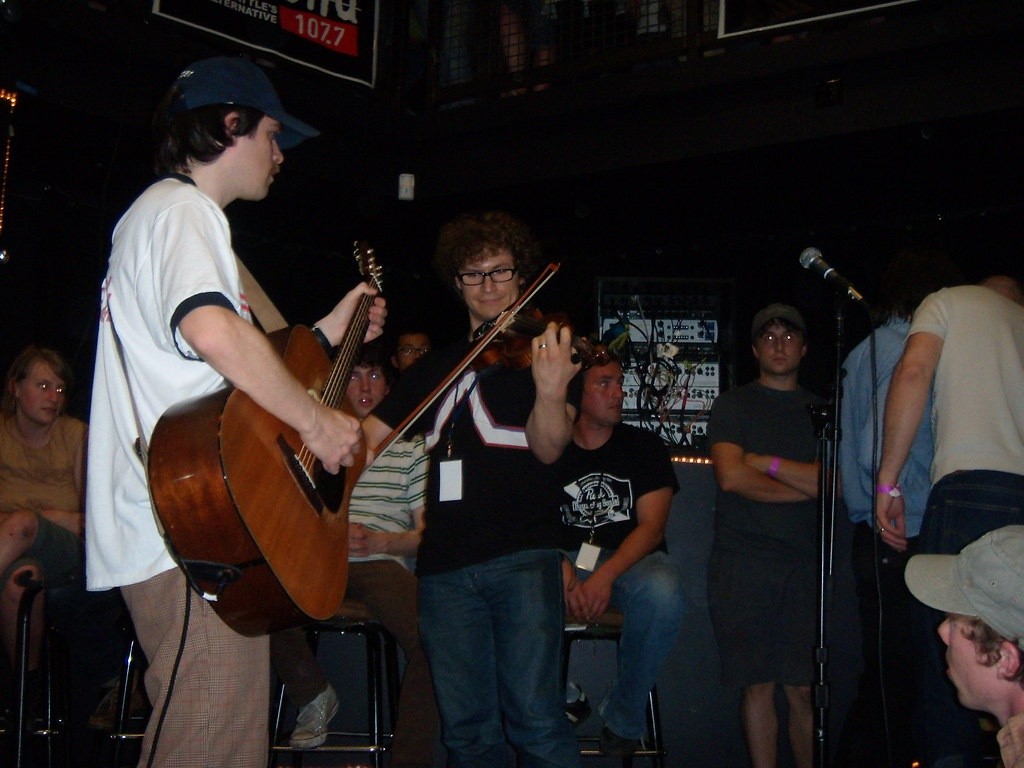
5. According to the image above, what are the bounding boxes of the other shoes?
[599,726,641,767]
[563,698,592,732]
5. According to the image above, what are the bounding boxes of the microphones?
[799,247,866,305]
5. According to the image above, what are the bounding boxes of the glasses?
[457,264,520,285]
[397,346,429,358]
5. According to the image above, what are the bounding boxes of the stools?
[268,618,403,768]
[0,619,72,768]
[87,610,152,768]
[559,609,671,768]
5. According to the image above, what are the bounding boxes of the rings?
[539,344,547,349]
[878,528,886,534]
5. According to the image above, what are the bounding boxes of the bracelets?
[876,482,902,497]
[767,455,781,476]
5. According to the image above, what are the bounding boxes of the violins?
[466,304,611,372]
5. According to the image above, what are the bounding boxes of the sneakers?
[287,682,342,750]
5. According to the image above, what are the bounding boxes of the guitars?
[145,235,386,640]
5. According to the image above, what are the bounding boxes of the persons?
[390,321,437,372]
[0,334,91,676]
[538,337,687,765]
[818,257,952,768]
[286,340,429,755]
[705,305,833,768]
[875,242,1024,768]
[902,524,1024,767]
[83,52,389,768]
[381,215,585,767]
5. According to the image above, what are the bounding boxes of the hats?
[749,304,805,342]
[165,57,321,151]
[906,524,1024,652]
